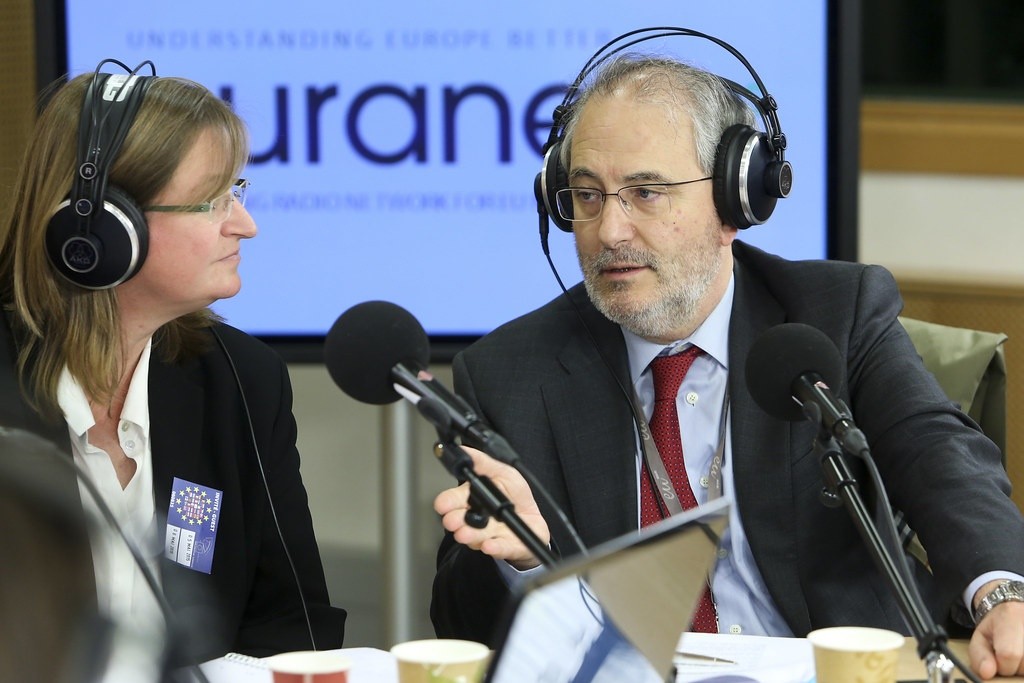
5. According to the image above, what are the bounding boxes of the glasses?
[552,173,713,222]
[143,178,251,224]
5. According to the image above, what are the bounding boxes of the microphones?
[321,300,518,466]
[745,323,870,460]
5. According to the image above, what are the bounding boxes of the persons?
[0,71,350,683]
[430,59,1024,683]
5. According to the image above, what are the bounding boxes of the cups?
[807,626,906,683]
[268,651,352,683]
[390,639,490,683]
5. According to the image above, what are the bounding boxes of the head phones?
[41,59,158,290]
[533,27,792,255]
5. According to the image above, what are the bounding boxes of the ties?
[640,346,717,633]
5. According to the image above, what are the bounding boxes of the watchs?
[975,582,1024,624]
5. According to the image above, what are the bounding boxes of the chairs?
[889,314,1010,625]
